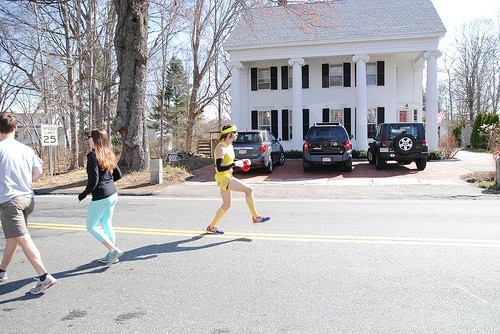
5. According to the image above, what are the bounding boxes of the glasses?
[87,135,92,140]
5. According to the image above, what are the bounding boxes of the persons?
[166,154,172,161]
[79,128,123,269]
[206,124,271,234]
[0,114,57,294]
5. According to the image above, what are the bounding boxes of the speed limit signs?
[42,124,58,146]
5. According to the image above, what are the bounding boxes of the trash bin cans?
[150,157,163,185]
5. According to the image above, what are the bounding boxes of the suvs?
[302,122,354,172]
[366,122,429,170]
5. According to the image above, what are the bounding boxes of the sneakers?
[253,214,271,223]
[106,247,122,267]
[102,252,120,263]
[30,274,58,295]
[207,226,224,234]
[0,272,8,282]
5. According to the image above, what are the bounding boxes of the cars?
[233,130,285,174]
